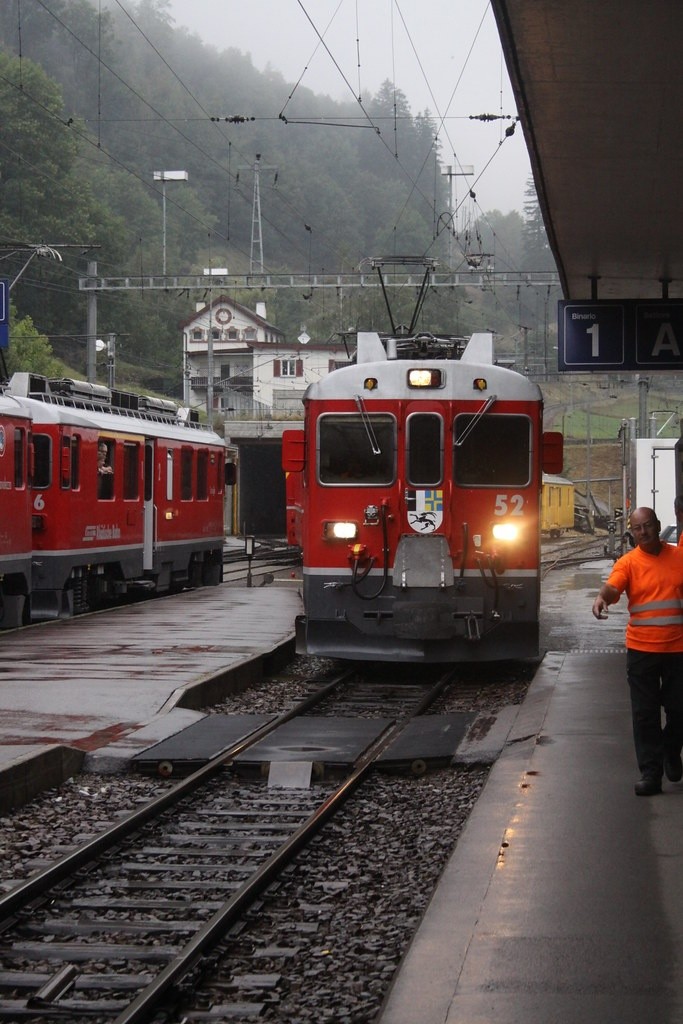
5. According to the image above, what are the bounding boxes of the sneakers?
[635,767,662,795]
[664,754,683,782]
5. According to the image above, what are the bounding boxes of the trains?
[1,364,237,633]
[280,253,567,681]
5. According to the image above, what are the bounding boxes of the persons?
[674,495,683,547]
[97,443,113,475]
[592,507,683,796]
